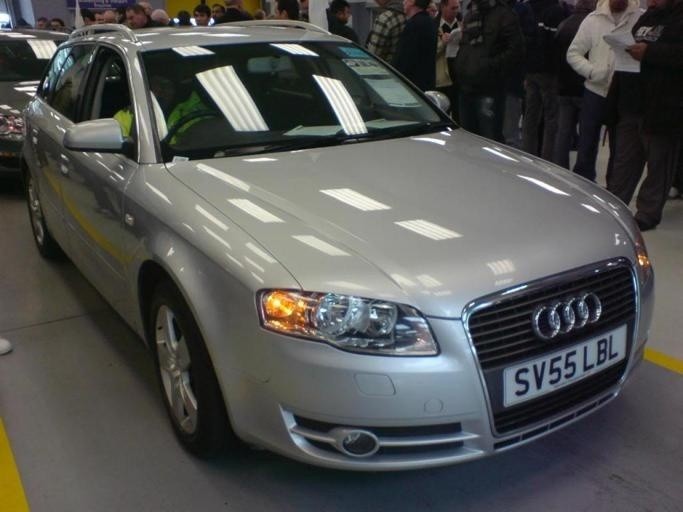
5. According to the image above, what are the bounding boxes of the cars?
[0,28,76,193]
[23,16,656,479]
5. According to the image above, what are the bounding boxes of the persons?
[327,1,683,230]
[56,45,89,112]
[18,16,65,31]
[0,338,13,355]
[81,1,309,86]
[112,63,212,143]
